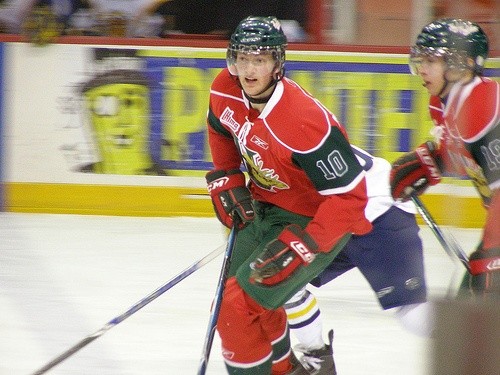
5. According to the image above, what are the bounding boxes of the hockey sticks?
[31,241,227,375]
[196,210,239,374]
[404,186,471,274]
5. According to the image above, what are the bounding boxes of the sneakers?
[287,345,335,374]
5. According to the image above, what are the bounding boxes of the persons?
[281,143,434,375]
[389,16,500,299]
[205,14,374,375]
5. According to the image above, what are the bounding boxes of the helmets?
[409,16,489,79]
[226,16,287,77]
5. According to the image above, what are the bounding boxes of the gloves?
[205,168,255,231]
[251,225,318,285]
[389,141,444,203]
